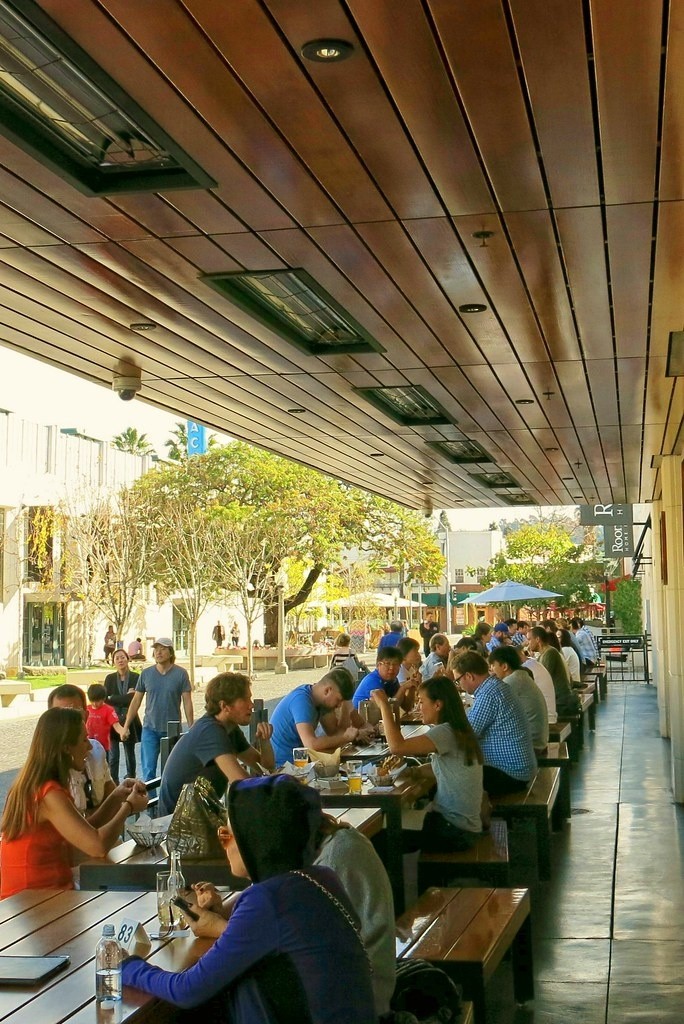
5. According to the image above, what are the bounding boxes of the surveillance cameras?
[422,508,433,518]
[112,377,141,401]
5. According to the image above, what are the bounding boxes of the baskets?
[126,826,167,847]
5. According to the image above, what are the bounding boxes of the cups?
[434,662,443,676]
[346,760,362,794]
[157,871,181,926]
[293,748,309,768]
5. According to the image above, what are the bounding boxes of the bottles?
[388,698,401,730]
[167,851,185,902]
[96,924,122,1011]
[424,619,428,624]
[367,690,380,726]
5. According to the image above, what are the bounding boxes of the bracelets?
[122,800,137,818]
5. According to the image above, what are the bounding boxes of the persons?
[210,618,242,647]
[46,685,125,867]
[157,671,277,818]
[96,773,397,1024]
[86,683,131,765]
[369,675,485,862]
[450,649,538,795]
[418,612,440,658]
[352,616,602,794]
[102,623,148,666]
[121,637,194,819]
[105,650,141,786]
[1,705,149,901]
[267,669,359,768]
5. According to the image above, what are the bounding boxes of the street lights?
[274,571,288,673]
[392,589,399,620]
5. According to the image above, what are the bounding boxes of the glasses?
[84,780,93,809]
[380,661,400,667]
[453,673,464,686]
[218,826,234,846]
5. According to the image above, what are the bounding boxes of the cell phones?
[174,896,200,922]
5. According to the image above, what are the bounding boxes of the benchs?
[378,654,608,1024]
[92,658,147,663]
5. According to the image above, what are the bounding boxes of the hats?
[150,638,174,648]
[494,623,509,632]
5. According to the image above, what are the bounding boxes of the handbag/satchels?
[342,648,358,682]
[167,777,227,863]
[390,958,461,1019]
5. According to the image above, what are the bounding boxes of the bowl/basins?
[313,766,340,780]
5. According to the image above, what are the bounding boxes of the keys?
[457,579,564,619]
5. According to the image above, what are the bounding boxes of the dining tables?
[260,762,437,921]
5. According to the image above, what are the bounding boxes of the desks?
[79,797,382,890]
[0,889,240,1024]
[312,722,433,762]
